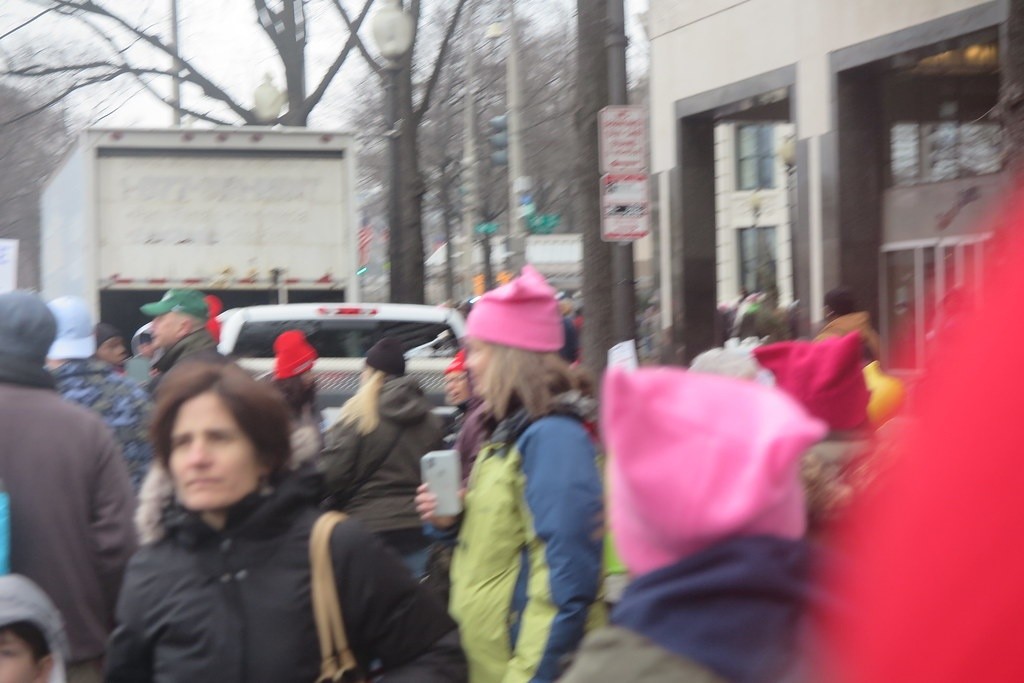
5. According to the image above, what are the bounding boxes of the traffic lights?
[489,114,511,165]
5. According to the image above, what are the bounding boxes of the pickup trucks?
[214,302,470,410]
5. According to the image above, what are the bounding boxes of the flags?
[358,216,374,262]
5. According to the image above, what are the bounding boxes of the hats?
[467,264,565,351]
[272,330,318,380]
[0,574,68,683]
[139,287,208,318]
[824,287,856,317]
[366,338,405,375]
[603,364,829,571]
[96,323,123,349]
[445,350,465,375]
[1,292,58,389]
[754,331,872,430]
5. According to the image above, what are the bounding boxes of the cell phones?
[420,449,462,516]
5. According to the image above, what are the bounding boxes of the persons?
[0,289,970,683]
[100,358,472,683]
[415,267,607,683]
[554,363,827,683]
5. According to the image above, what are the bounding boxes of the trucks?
[38,122,358,346]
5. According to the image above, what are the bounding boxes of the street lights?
[373,1,416,304]
